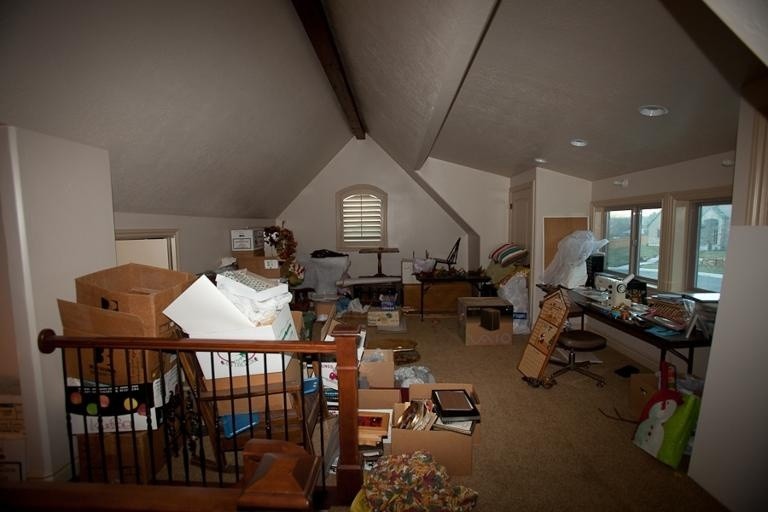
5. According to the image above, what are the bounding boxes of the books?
[320,383,340,414]
[394,399,436,431]
[686,288,715,333]
[350,406,387,468]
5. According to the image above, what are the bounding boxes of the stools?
[541,329,607,389]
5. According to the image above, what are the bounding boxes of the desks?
[567,289,711,376]
